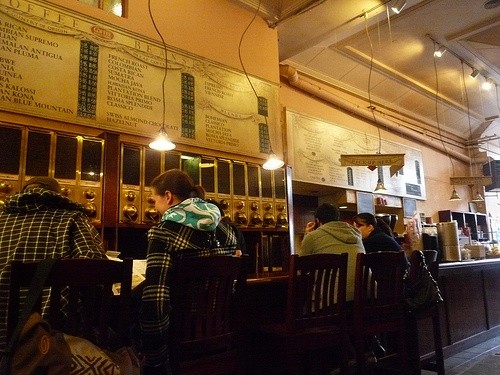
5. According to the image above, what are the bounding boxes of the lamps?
[237,0,285,170]
[148,0,177,152]
[362,1,485,203]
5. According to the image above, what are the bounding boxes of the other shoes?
[329,351,377,375]
[374,346,386,357]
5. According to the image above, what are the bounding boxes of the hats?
[22,177,61,193]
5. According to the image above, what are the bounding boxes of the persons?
[209,200,246,254]
[138,168,239,375]
[299,202,377,375]
[0,176,108,352]
[352,213,410,358]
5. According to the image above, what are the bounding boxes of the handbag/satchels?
[403,250,445,309]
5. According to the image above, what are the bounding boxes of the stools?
[0,252,443,375]
